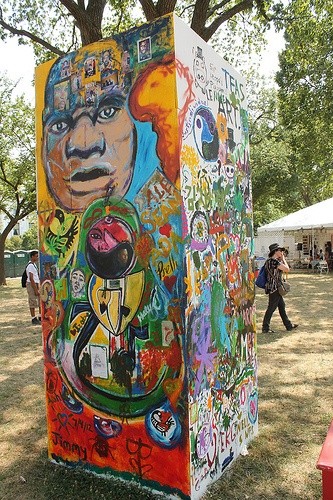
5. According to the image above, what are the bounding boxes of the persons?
[21,251,41,324]
[256,242,299,333]
[313,241,332,260]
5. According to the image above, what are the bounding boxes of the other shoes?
[38,314,41,322]
[262,329,274,333]
[32,317,41,325]
[290,324,298,329]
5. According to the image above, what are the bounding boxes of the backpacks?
[22,262,37,288]
[256,259,270,288]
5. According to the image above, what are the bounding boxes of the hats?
[268,243,285,257]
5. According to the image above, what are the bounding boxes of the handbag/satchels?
[277,281,290,296]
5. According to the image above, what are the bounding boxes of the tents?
[257,196,333,275]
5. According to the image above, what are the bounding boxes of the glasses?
[277,250,283,252]
